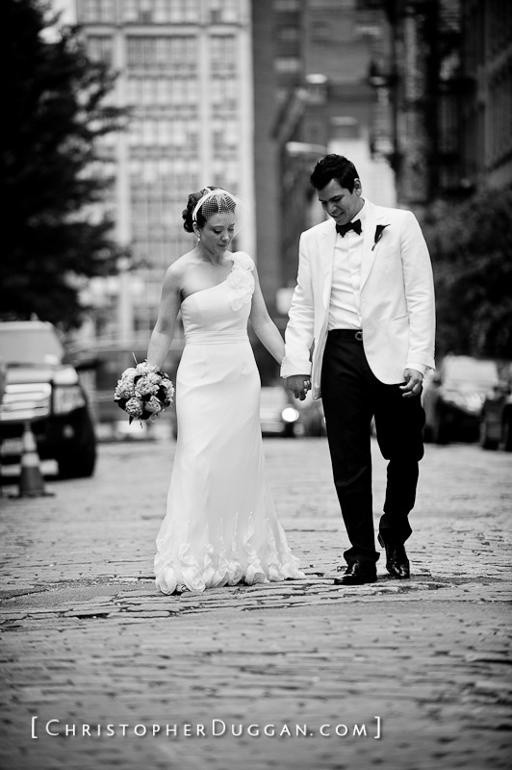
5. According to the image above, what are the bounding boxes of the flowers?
[113,360,176,426]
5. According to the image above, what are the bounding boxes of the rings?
[412,390,417,395]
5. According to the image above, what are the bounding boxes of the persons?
[279,154,437,586]
[145,183,311,595]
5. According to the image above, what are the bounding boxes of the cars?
[420,351,512,452]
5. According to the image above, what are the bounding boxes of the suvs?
[0,313,99,478]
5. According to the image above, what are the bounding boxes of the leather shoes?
[334,559,377,584]
[378,533,410,579]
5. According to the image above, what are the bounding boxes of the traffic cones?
[9,420,56,499]
